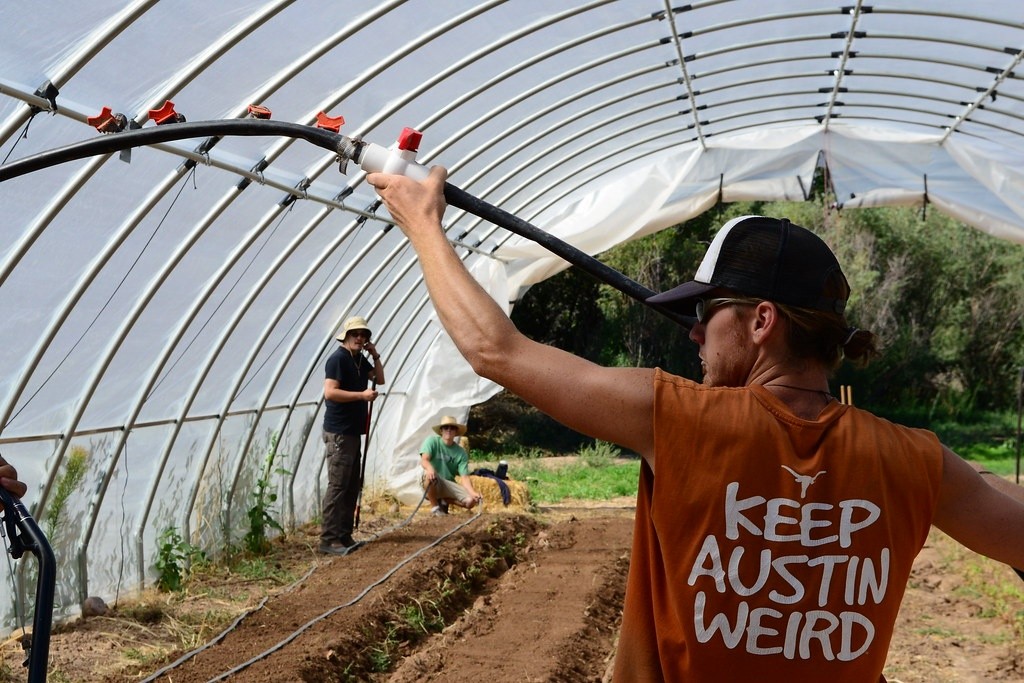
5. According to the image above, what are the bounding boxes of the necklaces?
[761,384,831,404]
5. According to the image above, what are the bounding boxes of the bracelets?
[978,471,993,474]
[373,353,381,360]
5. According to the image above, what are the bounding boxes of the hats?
[433,416,467,437]
[646,217,850,315]
[336,316,372,344]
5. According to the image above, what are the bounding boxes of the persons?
[367,166,1024,682]
[317,316,385,555]
[419,415,481,518]
[0,454,27,515]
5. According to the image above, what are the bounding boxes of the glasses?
[693,297,762,322]
[441,424,457,431]
[347,329,368,339]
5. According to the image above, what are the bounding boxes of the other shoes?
[432,499,449,516]
[321,535,362,558]
